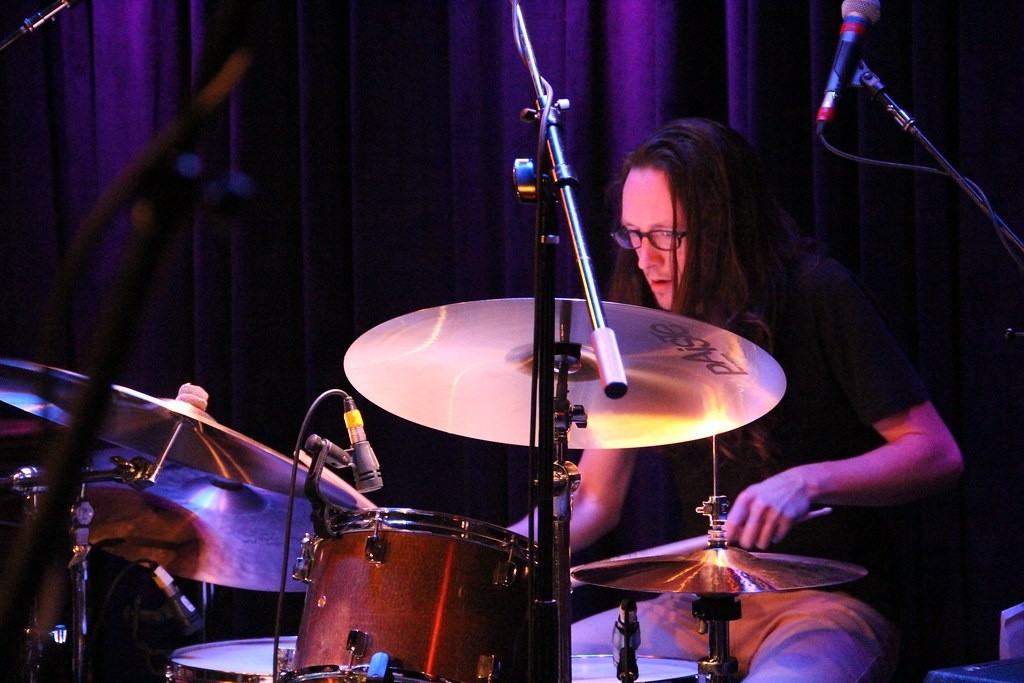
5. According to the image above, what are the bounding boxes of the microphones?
[816,0,880,135]
[611,600,641,669]
[343,397,384,494]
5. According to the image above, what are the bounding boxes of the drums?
[167,506,713,683]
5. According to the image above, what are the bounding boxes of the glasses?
[610,220,690,251]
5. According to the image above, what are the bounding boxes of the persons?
[504,112,967,683]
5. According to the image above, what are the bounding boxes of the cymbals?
[570,546,865,595]
[0,357,384,594]
[342,296,788,450]
